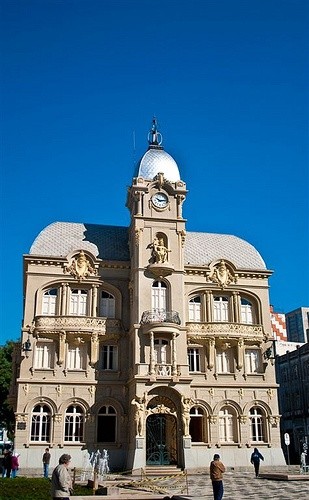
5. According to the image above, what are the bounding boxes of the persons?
[2,451,20,478]
[154,238,171,262]
[210,454,225,500]
[42,448,51,477]
[52,454,72,500]
[251,448,264,477]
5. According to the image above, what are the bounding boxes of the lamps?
[20,336,32,358]
[263,348,275,365]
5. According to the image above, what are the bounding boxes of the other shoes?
[256,476,258,478]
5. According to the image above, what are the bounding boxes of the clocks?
[151,192,169,207]
